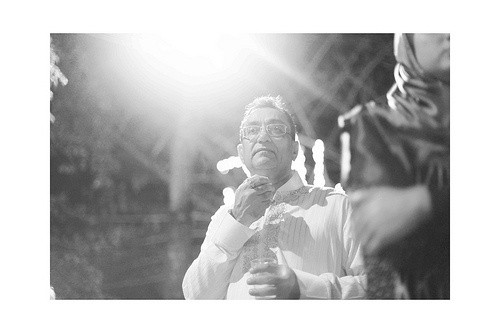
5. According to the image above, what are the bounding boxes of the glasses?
[240,123,293,140]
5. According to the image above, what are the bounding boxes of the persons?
[336,33,450,300]
[181,94,369,299]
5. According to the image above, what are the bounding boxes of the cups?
[250,258,278,299]
